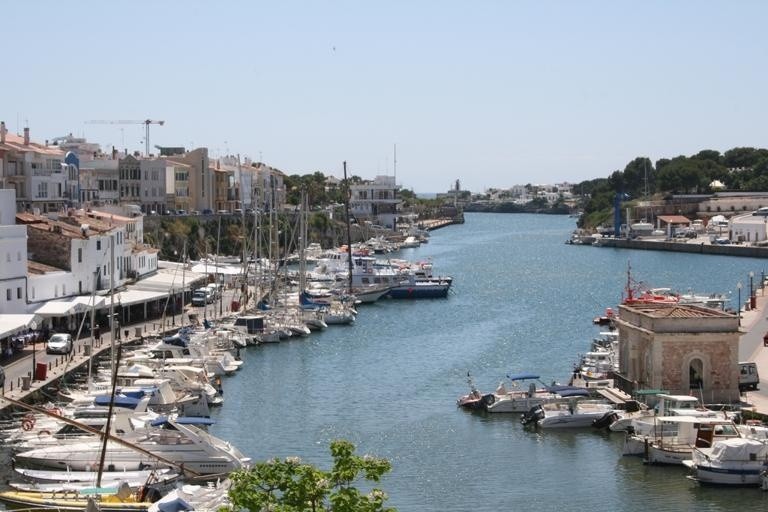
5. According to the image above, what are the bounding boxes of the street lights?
[737,281,742,326]
[750,271,754,310]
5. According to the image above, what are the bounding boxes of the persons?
[114,318,120,339]
[94,324,101,347]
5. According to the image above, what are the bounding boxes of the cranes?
[85,119,165,156]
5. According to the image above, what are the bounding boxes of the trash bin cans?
[83,345,90,356]
[21,377,31,390]
[36,363,47,380]
[135,328,141,337]
